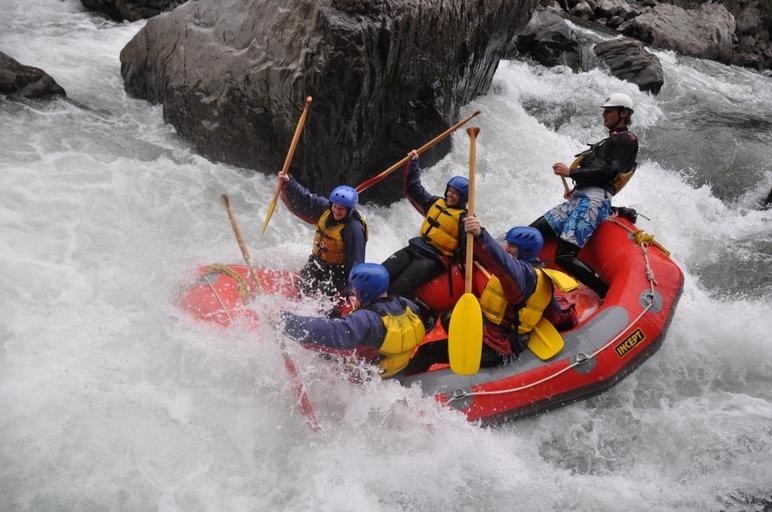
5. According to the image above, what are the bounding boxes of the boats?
[173,215,687,432]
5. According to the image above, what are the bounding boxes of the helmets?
[329,185,358,217]
[504,227,544,260]
[445,176,469,202]
[600,94,633,113]
[348,263,390,303]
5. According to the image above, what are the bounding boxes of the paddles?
[448,128,483,375]
[474,259,564,361]
[354,110,482,194]
[253,96,313,242]
[220,193,322,434]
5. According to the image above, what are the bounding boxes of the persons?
[278,172,367,317]
[262,262,427,386]
[402,215,555,374]
[526,92,638,307]
[381,149,476,298]
